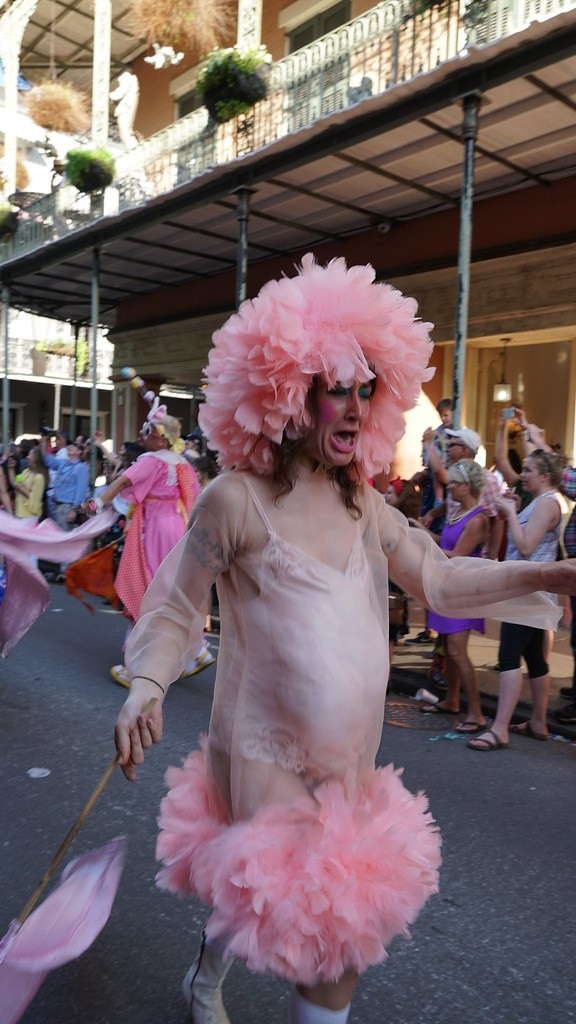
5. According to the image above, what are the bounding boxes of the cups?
[415,688,439,704]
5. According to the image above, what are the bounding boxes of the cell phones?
[503,407,516,421]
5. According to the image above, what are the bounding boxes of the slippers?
[420,702,461,715]
[510,722,549,740]
[466,730,510,751]
[454,717,488,733]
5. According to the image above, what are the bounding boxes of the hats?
[447,458,486,489]
[146,415,182,446]
[444,426,481,454]
[67,440,87,449]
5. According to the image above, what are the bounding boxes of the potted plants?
[64,146,117,194]
[0,202,21,241]
[196,46,276,125]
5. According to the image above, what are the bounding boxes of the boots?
[179,925,234,1024]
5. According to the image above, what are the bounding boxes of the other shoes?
[553,704,576,722]
[560,686,576,700]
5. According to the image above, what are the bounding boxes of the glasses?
[455,463,470,483]
[443,442,465,449]
[533,450,545,456]
[141,423,150,438]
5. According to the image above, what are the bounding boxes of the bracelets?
[73,496,104,519]
[133,676,165,694]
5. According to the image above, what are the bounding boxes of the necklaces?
[448,502,480,525]
[516,488,557,519]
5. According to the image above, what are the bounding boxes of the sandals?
[111,664,134,689]
[180,647,217,679]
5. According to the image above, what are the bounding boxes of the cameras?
[40,426,57,437]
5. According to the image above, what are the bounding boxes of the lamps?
[492,336,512,402]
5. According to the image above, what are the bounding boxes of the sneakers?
[405,630,438,646]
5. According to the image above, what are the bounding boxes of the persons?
[401,399,453,645]
[488,405,576,724]
[468,449,568,750]
[97,442,144,610]
[421,427,506,561]
[384,477,421,691]
[76,415,216,693]
[0,392,89,607]
[182,431,216,495]
[408,458,507,732]
[113,250,576,1024]
